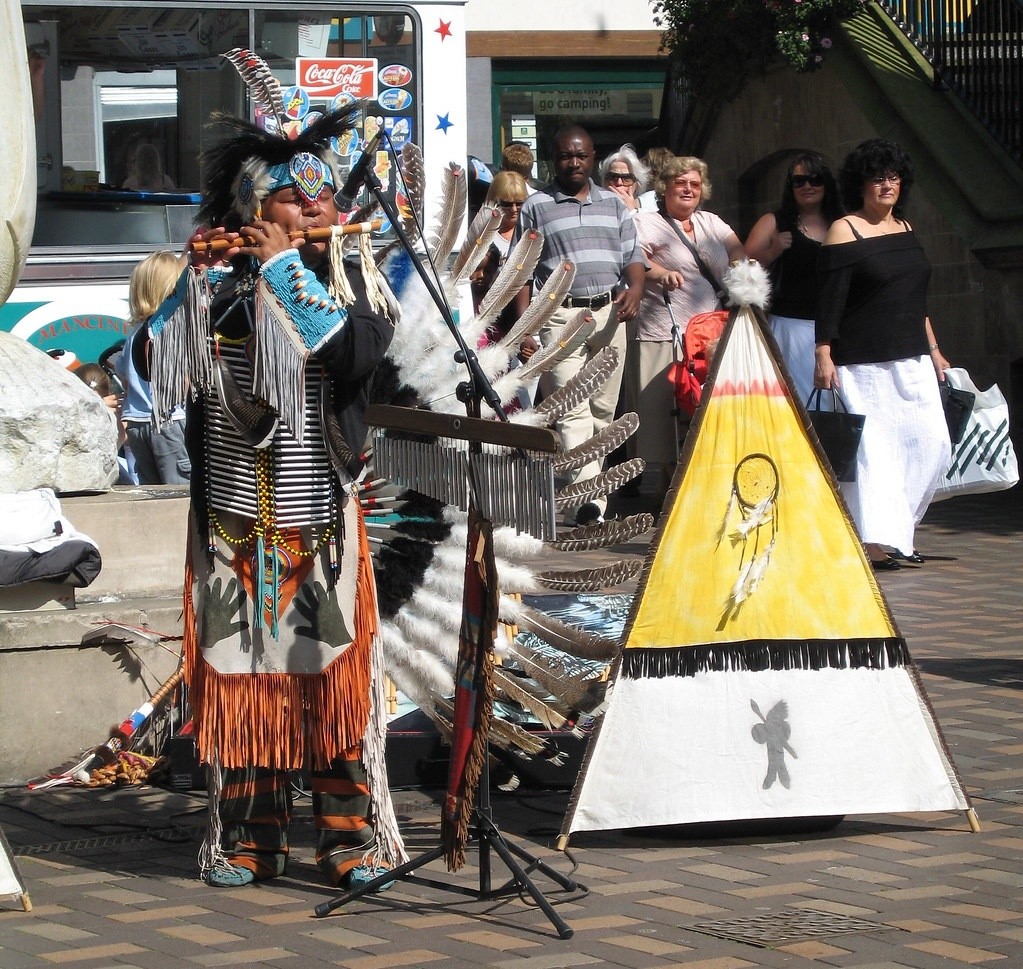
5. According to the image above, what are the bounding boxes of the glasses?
[866,175,902,185]
[497,199,524,207]
[790,174,824,188]
[606,172,635,183]
[672,179,701,190]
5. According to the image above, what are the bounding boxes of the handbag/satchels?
[937,372,975,443]
[929,368,1019,504]
[804,379,866,482]
[763,212,784,318]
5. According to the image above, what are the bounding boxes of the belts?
[535,277,624,312]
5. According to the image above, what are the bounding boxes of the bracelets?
[930,344,939,351]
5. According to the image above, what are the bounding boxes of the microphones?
[333,130,387,214]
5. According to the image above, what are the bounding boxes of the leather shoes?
[870,556,901,570]
[895,547,924,563]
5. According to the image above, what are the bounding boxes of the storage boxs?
[0,581,76,615]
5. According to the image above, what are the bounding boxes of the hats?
[194,47,370,233]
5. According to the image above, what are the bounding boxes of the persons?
[65,249,191,490]
[463,123,949,566]
[124,142,174,190]
[134,145,413,896]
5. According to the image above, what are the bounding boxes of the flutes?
[191,221,381,251]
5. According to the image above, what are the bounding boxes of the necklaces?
[797,215,816,240]
[870,220,895,233]
[683,225,694,232]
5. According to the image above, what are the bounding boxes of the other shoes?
[207,864,256,887]
[345,867,395,892]
[620,479,642,498]
[563,500,606,530]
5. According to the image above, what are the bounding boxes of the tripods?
[313,169,578,940]
